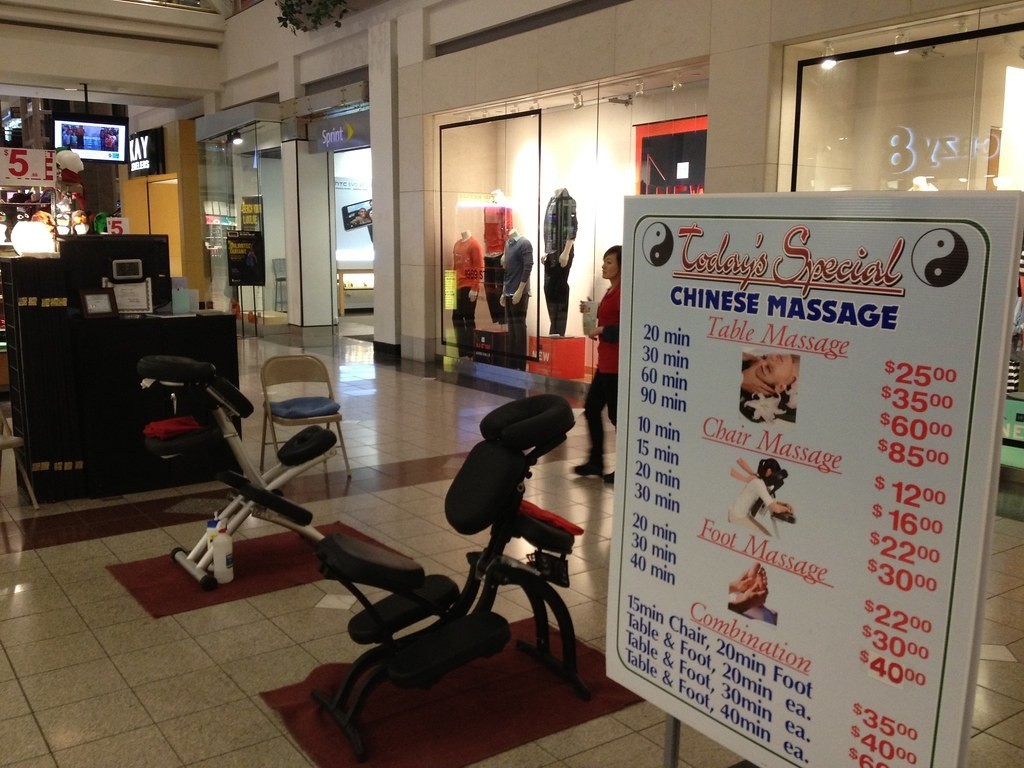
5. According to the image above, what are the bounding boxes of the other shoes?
[603,471,616,483]
[574,461,604,476]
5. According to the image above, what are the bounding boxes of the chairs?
[168,361,337,590]
[309,392,592,762]
[259,354,353,478]
[0,406,39,511]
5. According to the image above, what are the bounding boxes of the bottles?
[213,528,233,583]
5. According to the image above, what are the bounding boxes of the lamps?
[529,100,540,118]
[821,40,837,70]
[958,18,970,43]
[893,34,911,55]
[511,103,519,114]
[481,109,488,117]
[225,130,243,145]
[572,90,583,109]
[633,79,644,96]
[671,76,682,91]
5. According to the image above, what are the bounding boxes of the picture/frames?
[79,287,120,318]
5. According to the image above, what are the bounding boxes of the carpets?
[106,520,413,618]
[259,617,646,768]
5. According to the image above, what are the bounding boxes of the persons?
[351,201,373,225]
[572,245,621,483]
[740,350,800,410]
[499,229,534,372]
[540,187,577,339]
[62,124,118,151]
[730,458,794,537]
[729,563,778,626]
[482,189,514,327]
[451,231,484,361]
